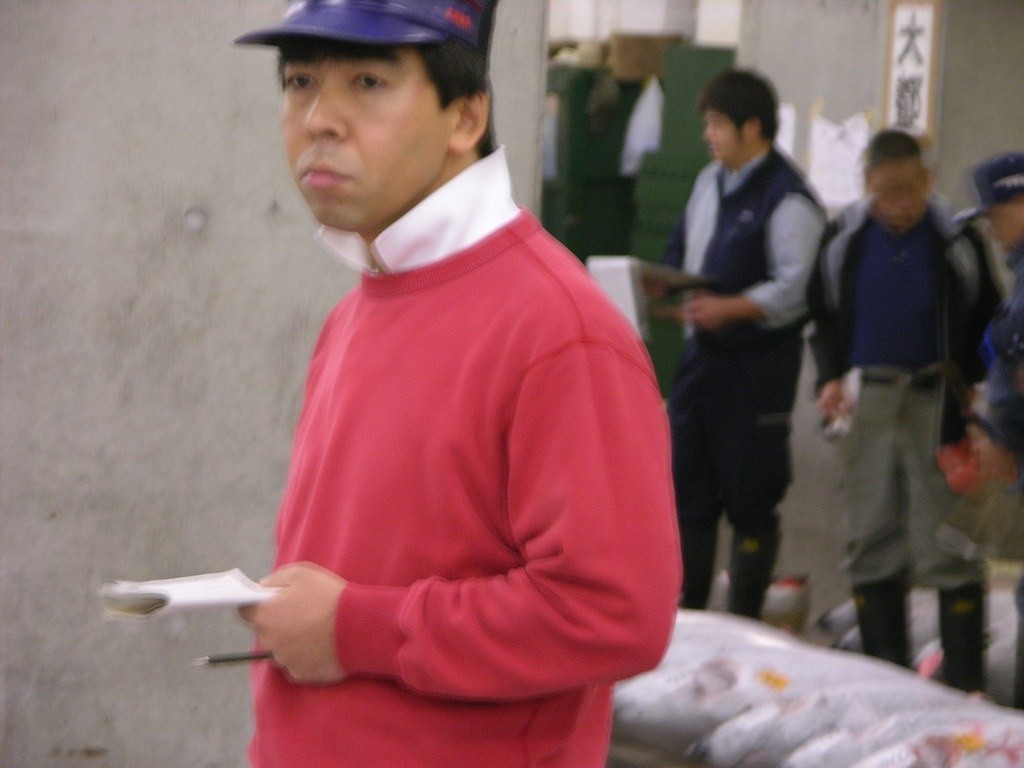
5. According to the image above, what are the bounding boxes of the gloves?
[938,444,982,498]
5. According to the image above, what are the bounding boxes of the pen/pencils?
[198,646,274,669]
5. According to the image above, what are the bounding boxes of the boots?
[675,525,714,608]
[728,525,780,621]
[852,567,909,669]
[926,582,983,691]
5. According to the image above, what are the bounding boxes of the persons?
[636,67,826,621]
[805,131,1003,698]
[236,1,684,767]
[950,150,1024,713]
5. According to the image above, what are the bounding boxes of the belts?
[859,375,949,392]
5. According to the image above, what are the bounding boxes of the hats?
[233,0,496,45]
[950,149,1024,223]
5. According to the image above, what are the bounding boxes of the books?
[98,568,276,623]
